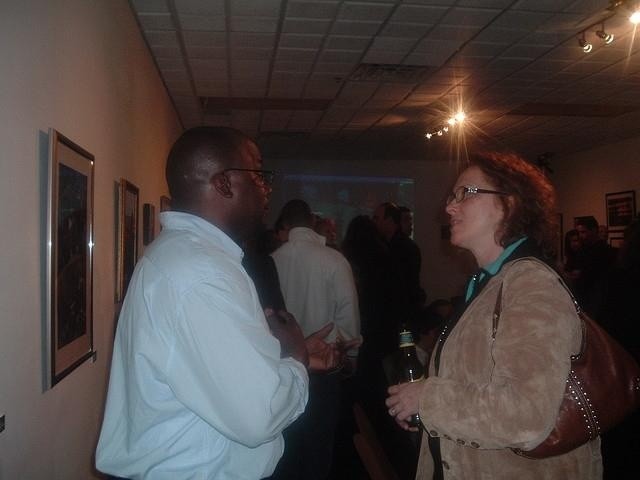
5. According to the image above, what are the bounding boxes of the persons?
[385,148,603,480]
[565,209,640,345]
[265,198,433,480]
[93,126,360,480]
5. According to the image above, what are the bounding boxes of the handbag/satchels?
[510,311,639,460]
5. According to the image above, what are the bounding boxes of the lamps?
[578,21,616,54]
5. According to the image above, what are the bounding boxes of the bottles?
[396,326,428,427]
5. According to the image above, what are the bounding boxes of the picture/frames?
[540,213,563,266]
[605,190,637,248]
[42,124,170,390]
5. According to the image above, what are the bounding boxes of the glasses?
[223,168,275,185]
[448,186,509,203]
